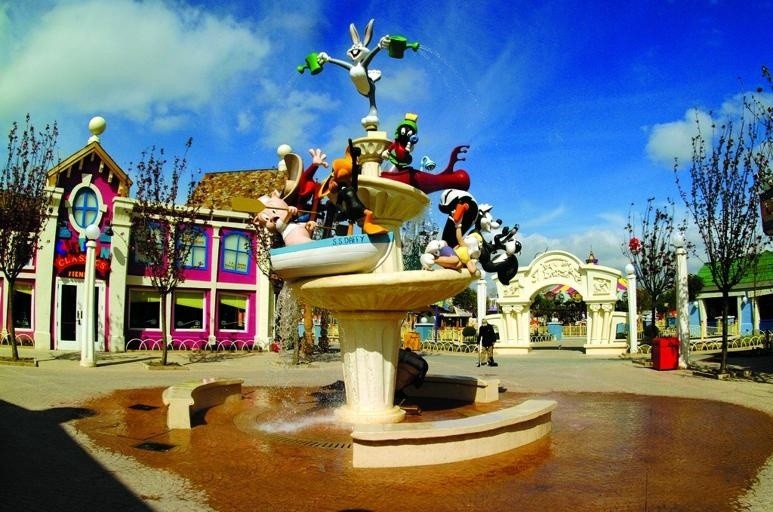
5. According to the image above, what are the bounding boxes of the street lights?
[672,233,691,369]
[477,270,488,336]
[78,224,101,368]
[625,264,638,354]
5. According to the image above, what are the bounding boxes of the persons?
[477,318,496,365]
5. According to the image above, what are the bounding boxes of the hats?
[482,319,487,326]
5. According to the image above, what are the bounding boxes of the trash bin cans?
[653,337,679,370]
[405,333,421,351]
[415,324,434,340]
[547,323,562,340]
